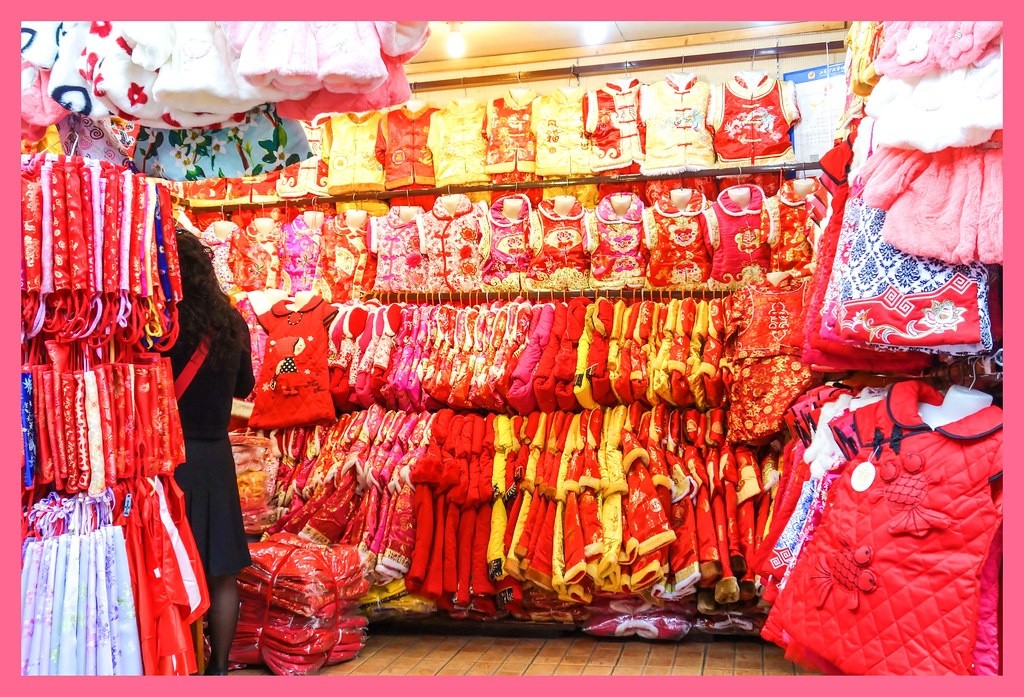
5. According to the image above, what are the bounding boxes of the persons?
[164,227,255,676]
